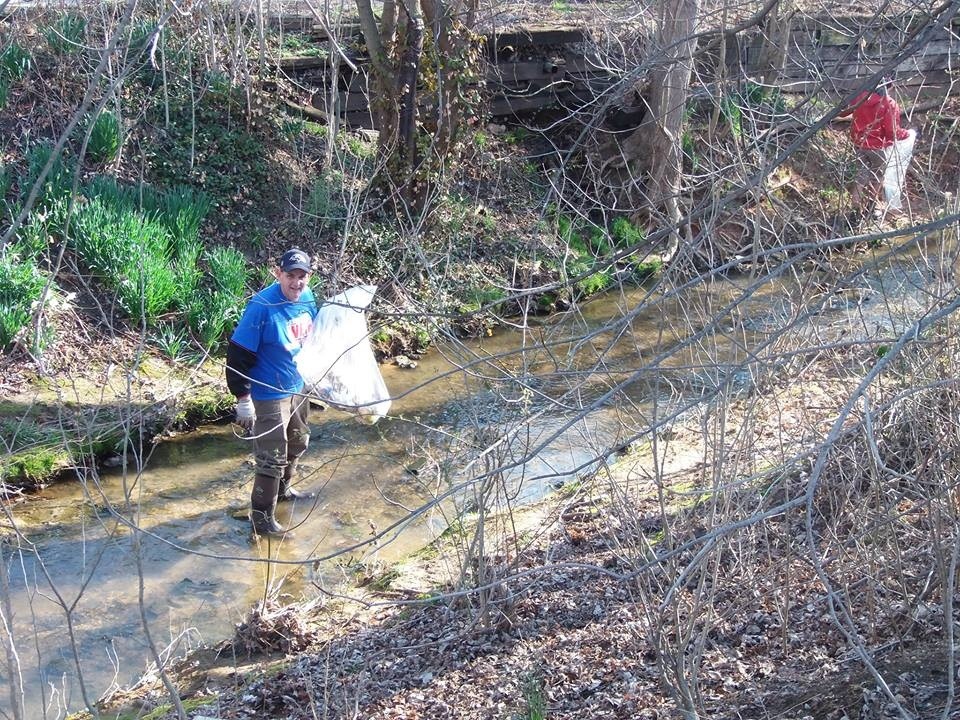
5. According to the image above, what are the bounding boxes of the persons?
[831,70,909,225]
[226,250,319,541]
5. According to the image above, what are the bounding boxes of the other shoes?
[874,212,882,220]
[849,211,862,228]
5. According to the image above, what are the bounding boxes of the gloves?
[236,399,257,431]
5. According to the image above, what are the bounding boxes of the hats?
[280,249,310,273]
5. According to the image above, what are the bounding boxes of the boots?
[251,474,295,538]
[278,455,316,502]
